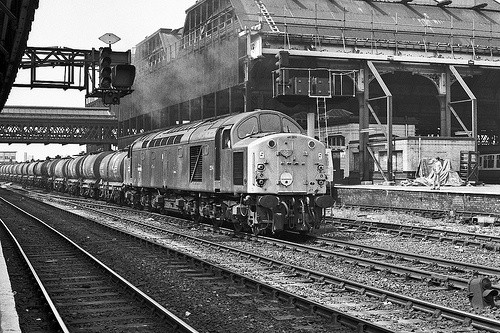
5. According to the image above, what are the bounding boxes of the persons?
[431,157,442,190]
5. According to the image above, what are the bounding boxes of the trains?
[1,110,337,236]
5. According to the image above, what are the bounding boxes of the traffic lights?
[98,46,136,104]
